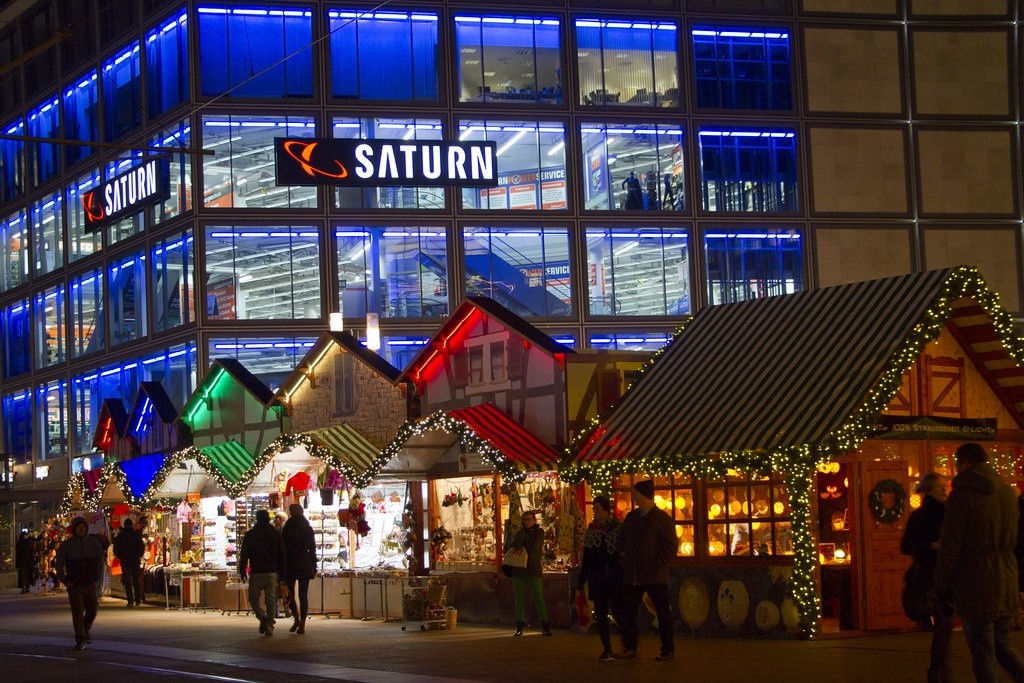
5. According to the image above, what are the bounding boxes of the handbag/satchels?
[558,487,585,552]
[320,488,333,505]
[269,492,282,508]
[187,493,200,503]
[502,546,528,569]
[339,509,349,527]
[402,513,409,529]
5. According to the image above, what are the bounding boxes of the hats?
[223,501,236,559]
[634,480,653,499]
[338,530,349,559]
[337,551,348,563]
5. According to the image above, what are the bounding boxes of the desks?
[491,93,559,101]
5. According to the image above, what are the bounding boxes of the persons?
[338,530,347,546]
[240,511,282,636]
[511,511,552,636]
[281,504,318,634]
[663,174,675,209]
[938,442,1024,683]
[392,521,406,536]
[622,172,642,200]
[55,517,105,650]
[338,551,347,566]
[114,519,145,607]
[900,473,974,683]
[621,481,678,660]
[273,512,293,617]
[15,532,35,593]
[579,496,637,661]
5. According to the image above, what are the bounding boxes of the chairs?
[478,86,560,100]
[583,89,620,105]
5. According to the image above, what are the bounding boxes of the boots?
[27,585,32,592]
[541,620,552,636]
[514,621,524,636]
[21,585,27,594]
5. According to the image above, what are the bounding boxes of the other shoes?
[599,651,615,662]
[297,628,305,634]
[126,601,140,607]
[290,620,300,632]
[260,616,268,634]
[621,651,639,657]
[265,632,273,636]
[656,653,675,661]
[75,643,85,650]
[87,633,91,642]
